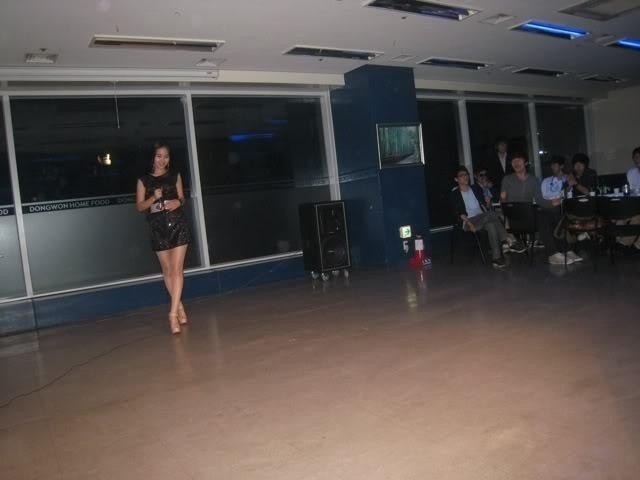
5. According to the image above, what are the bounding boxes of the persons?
[627,147,640,250]
[447,135,597,273]
[136,139,193,334]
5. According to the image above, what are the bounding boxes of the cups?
[566,185,620,199]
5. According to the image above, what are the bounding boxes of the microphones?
[155,185,163,204]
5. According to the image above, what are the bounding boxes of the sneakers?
[549,251,584,264]
[606,242,640,255]
[502,239,527,255]
[527,239,544,248]
[492,258,506,268]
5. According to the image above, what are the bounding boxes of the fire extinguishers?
[411,234,425,265]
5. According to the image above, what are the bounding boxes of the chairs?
[449,196,640,274]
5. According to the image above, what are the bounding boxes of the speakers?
[298,200,352,272]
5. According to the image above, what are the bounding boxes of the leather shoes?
[169,302,187,335]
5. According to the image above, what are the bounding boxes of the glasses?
[456,173,469,178]
